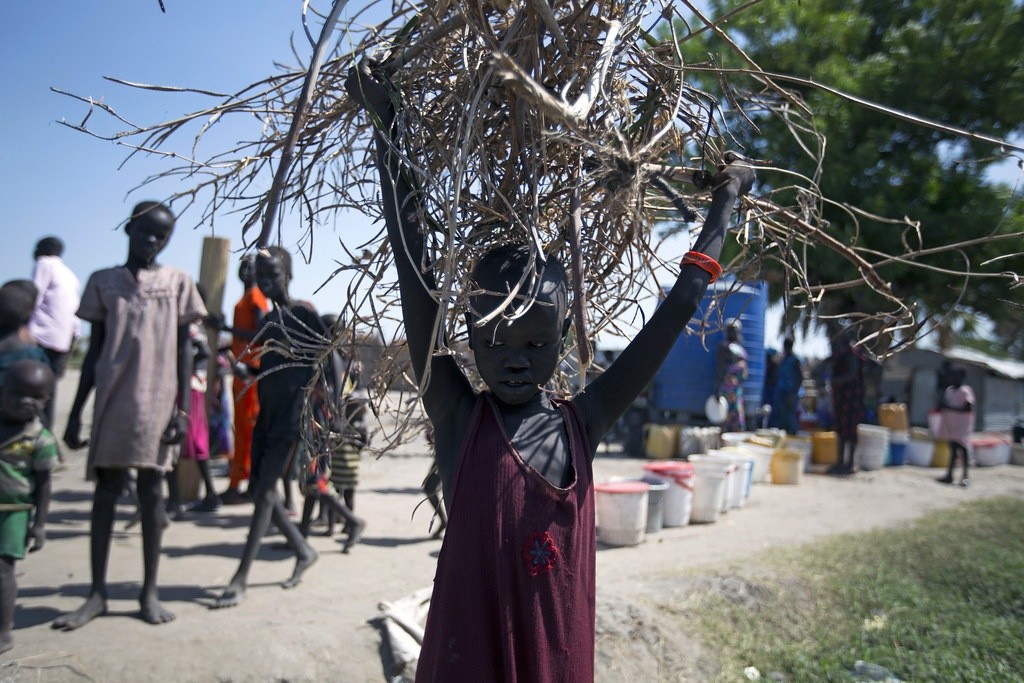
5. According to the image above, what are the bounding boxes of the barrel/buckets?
[857,422,909,471]
[642,460,695,527]
[773,451,800,485]
[814,431,837,464]
[907,440,934,467]
[688,454,733,514]
[626,477,671,533]
[785,435,814,471]
[643,424,721,458]
[596,482,649,546]
[709,449,755,509]
[722,429,784,482]
[935,443,951,467]
[968,436,1013,466]
[692,475,724,522]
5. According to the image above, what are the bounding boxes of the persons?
[26,237,81,464]
[766,339,803,435]
[53,202,208,628]
[165,247,367,608]
[715,318,747,431]
[426,423,448,539]
[825,334,866,476]
[935,365,974,488]
[760,348,783,427]
[0,280,63,467]
[344,57,757,683]
[599,348,616,372]
[0,360,57,654]
